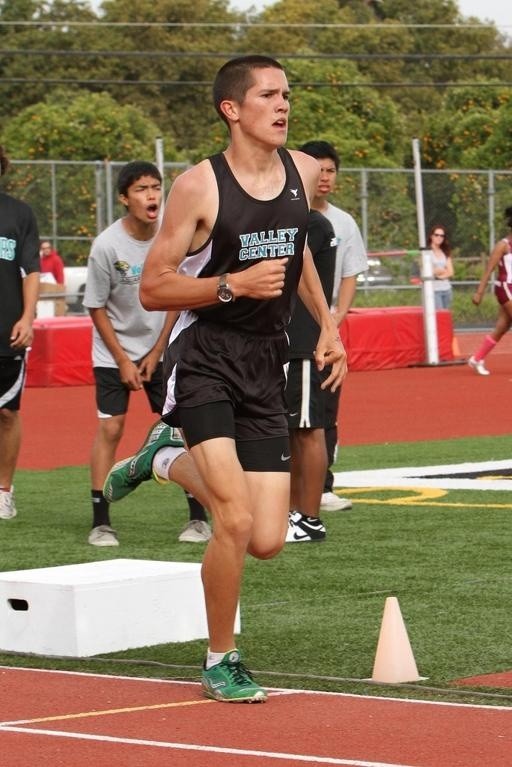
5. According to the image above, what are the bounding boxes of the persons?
[0,147,40,520]
[40,239,64,283]
[286,209,349,543]
[420,224,461,356]
[298,140,371,514]
[466,206,512,376]
[82,160,211,548]
[103,55,348,703]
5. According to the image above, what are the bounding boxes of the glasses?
[433,233,445,237]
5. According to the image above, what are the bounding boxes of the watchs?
[216,273,235,305]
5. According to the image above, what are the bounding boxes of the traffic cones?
[363,590,428,686]
[450,336,462,357]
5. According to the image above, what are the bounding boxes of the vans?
[355,252,423,284]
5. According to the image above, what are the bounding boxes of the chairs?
[67,284,86,314]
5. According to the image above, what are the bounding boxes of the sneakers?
[1,484,18,520]
[284,510,326,543]
[102,418,184,505]
[179,518,212,543]
[468,356,490,376]
[88,525,120,547]
[201,648,268,703]
[320,491,353,511]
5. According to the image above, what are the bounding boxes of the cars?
[58,264,90,316]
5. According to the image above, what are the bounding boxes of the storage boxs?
[0,554,241,658]
